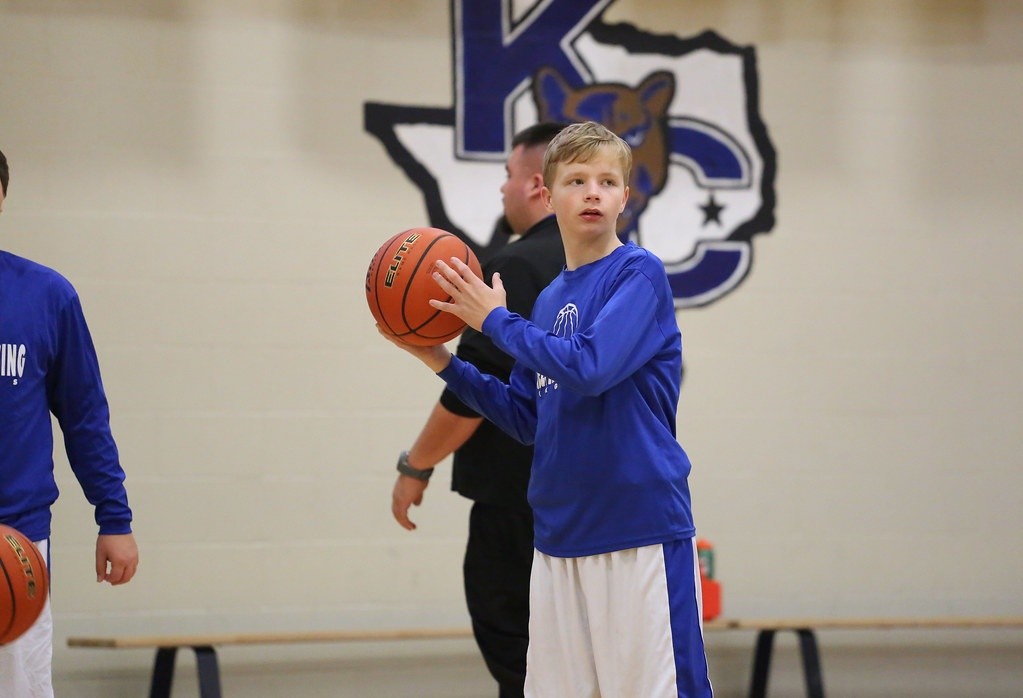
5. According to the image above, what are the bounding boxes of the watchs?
[396,450,433,479]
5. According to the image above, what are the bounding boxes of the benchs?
[64,615,1022,698]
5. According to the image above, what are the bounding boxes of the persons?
[374,123,715,698]
[0,151,139,698]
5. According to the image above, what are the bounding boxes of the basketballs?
[1,522,50,648]
[365,226,487,350]
